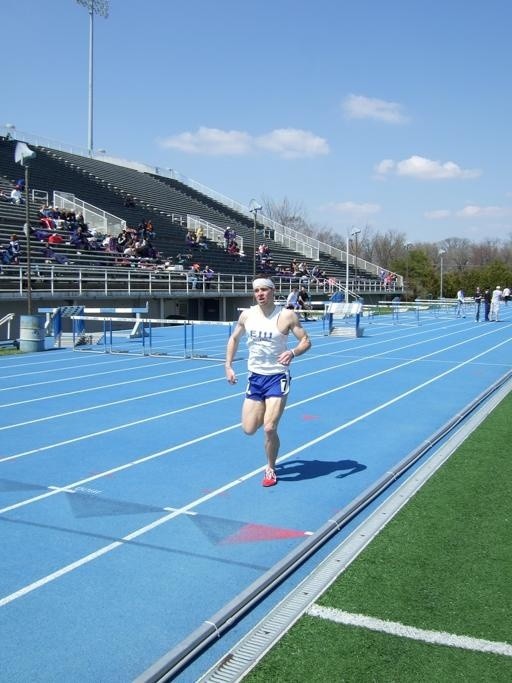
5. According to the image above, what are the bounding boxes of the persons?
[0,176,218,292]
[222,226,337,289]
[379,269,398,291]
[472,286,482,322]
[502,284,511,306]
[295,285,310,320]
[286,287,302,310]
[484,284,491,320]
[455,286,466,318]
[328,287,343,301]
[225,272,312,487]
[489,285,504,321]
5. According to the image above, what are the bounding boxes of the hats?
[496,285,503,289]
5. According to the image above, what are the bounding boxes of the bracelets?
[289,348,296,358]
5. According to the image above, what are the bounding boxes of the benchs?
[0,135,402,289]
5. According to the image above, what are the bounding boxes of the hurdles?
[37,307,242,360]
[238,297,486,337]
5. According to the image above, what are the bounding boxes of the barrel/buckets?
[19,314,45,352]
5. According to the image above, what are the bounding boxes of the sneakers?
[263,464,277,487]
